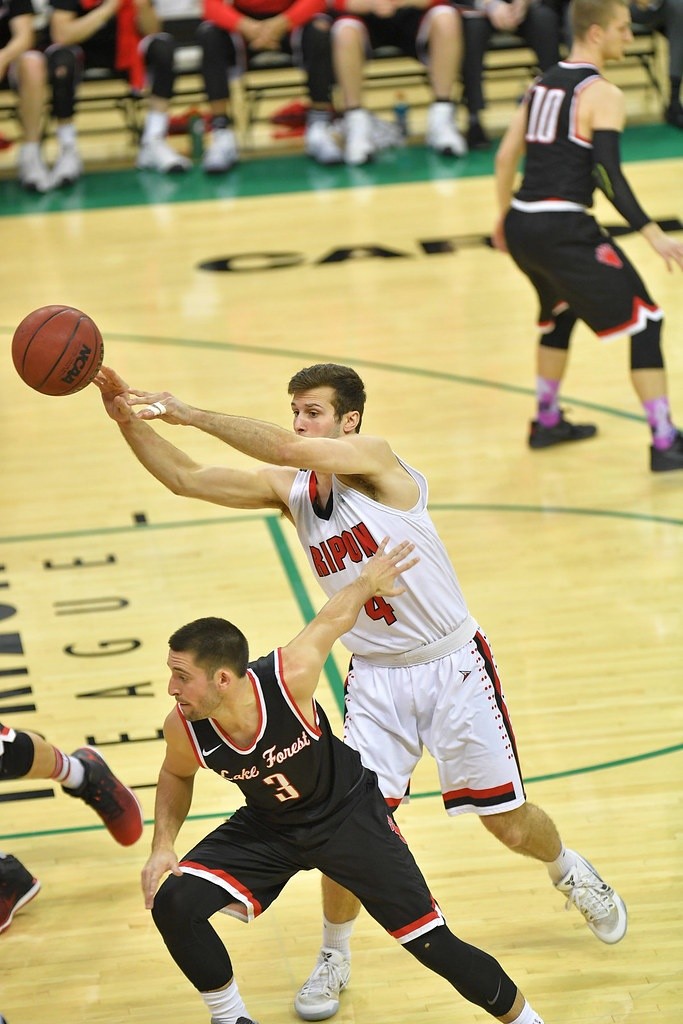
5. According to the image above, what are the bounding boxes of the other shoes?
[665,103,683,131]
[466,125,492,149]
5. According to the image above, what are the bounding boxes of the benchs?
[0,37,671,175]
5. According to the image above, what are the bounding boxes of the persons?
[490,0,682,472]
[142,535,547,1024]
[0,720,145,935]
[91,363,629,1021]
[0,0,681,196]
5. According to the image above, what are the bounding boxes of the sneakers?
[293,946,352,1021]
[303,122,343,164]
[50,146,85,186]
[61,746,144,847]
[424,101,468,156]
[552,846,629,946]
[17,151,51,193]
[0,855,41,935]
[341,110,377,166]
[199,128,240,174]
[650,430,683,471]
[133,134,194,175]
[529,409,596,448]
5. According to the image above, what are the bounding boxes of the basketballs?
[12,304,104,395]
